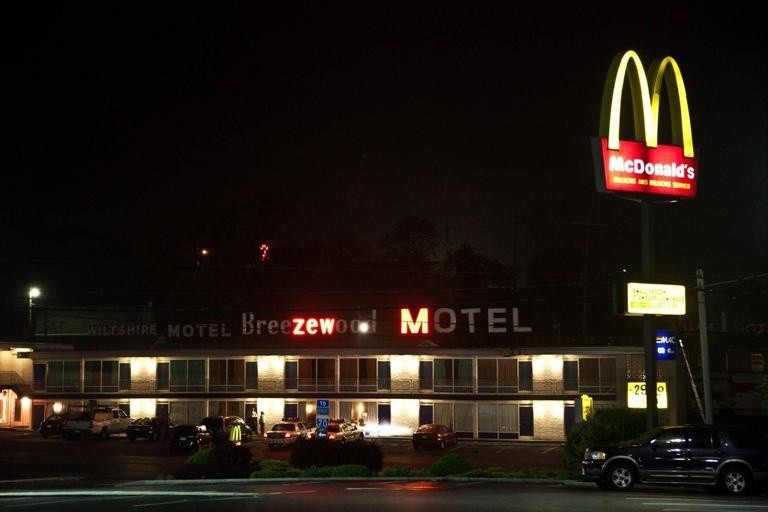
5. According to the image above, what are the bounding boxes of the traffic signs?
[316,416,329,432]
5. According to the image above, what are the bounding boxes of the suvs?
[582,423,752,496]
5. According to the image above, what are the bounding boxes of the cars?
[264,417,362,449]
[39,406,251,457]
[412,424,456,450]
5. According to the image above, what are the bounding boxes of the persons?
[249,407,257,417]
[259,411,266,435]
